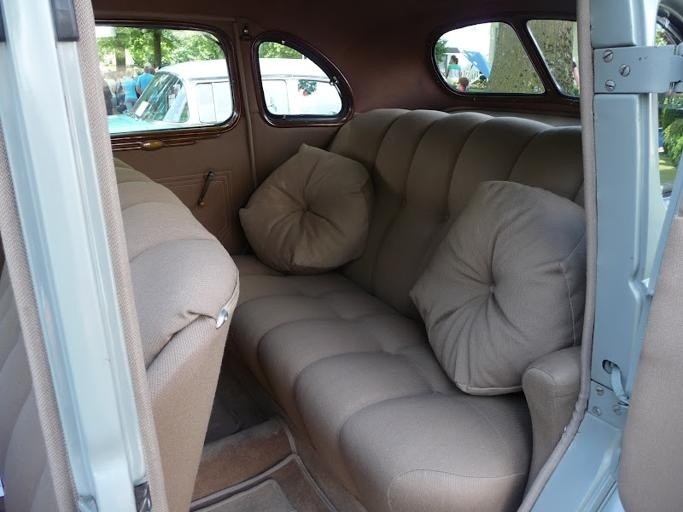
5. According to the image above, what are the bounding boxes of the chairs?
[1,156,241,512]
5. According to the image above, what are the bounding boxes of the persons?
[101,65,157,115]
[444,55,470,92]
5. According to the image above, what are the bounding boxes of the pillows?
[406,178,589,397]
[237,140,374,275]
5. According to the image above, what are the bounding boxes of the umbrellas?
[463,47,489,80]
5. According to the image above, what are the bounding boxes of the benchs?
[231,107,589,511]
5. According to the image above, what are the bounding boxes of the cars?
[106,58,341,133]
